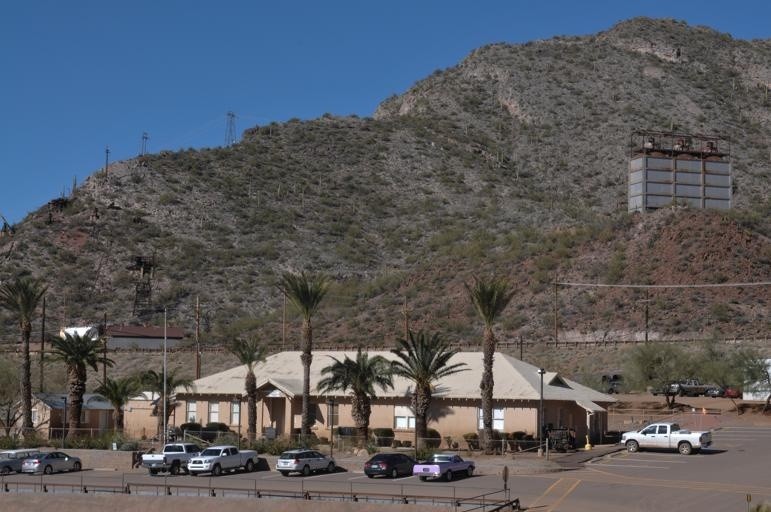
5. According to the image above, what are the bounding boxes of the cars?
[647,371,742,403]
[362,452,420,479]
[601,374,632,394]
[21,451,82,476]
[0,449,40,477]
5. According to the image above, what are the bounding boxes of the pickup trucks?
[185,445,258,478]
[412,453,475,482]
[140,442,203,477]
[619,421,712,456]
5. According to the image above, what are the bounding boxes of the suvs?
[274,449,336,478]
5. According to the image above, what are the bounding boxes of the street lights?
[537,367,546,449]
